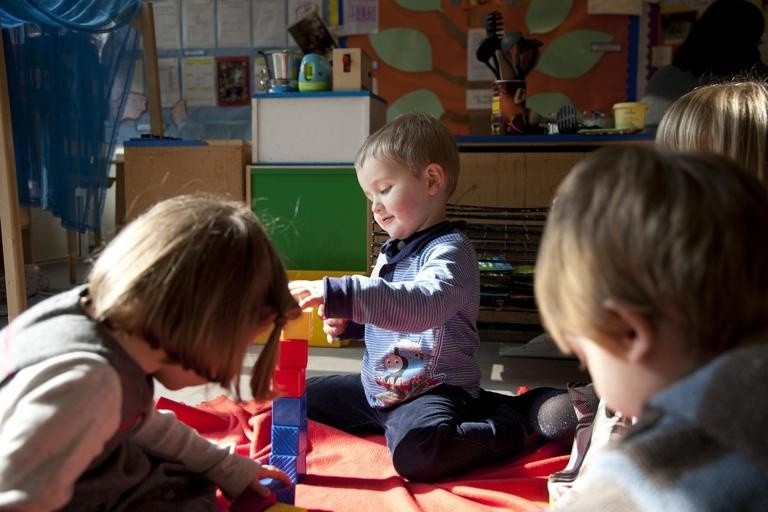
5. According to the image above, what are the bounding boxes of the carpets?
[149,392,572,512]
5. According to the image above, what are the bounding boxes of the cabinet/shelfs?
[370,135,655,344]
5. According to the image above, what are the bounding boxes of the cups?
[612,103,648,131]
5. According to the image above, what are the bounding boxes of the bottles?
[372,60,378,94]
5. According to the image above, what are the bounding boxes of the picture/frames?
[215,56,252,106]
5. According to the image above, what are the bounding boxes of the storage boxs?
[243,165,371,277]
[122,138,251,230]
[250,92,385,166]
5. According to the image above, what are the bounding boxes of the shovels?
[513,48,541,80]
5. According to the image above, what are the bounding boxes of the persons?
[285,111,569,485]
[0,190,302,512]
[643,0,768,111]
[532,137,768,508]
[651,78,768,196]
